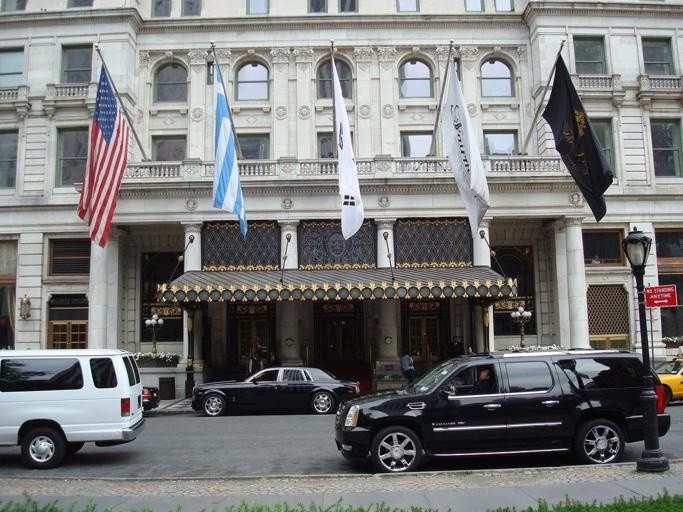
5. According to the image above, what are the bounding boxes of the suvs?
[334,345,672,474]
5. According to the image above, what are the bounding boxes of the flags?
[211,63,249,241]
[331,56,364,240]
[76,62,129,249]
[439,61,490,240]
[542,53,614,224]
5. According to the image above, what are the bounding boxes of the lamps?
[480,230,508,280]
[19,294,31,320]
[166,235,194,288]
[383,232,395,285]
[279,233,292,285]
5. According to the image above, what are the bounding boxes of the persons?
[466,345,475,355]
[400,349,416,382]
[248,351,264,376]
[267,350,280,367]
[447,335,464,358]
[467,369,492,395]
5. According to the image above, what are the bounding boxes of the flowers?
[661,336,683,342]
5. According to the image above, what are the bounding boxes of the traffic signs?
[645,285,676,308]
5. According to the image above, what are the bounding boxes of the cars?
[190,366,360,416]
[651,357,682,405]
[141,386,160,413]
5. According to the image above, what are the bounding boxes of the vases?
[665,341,679,348]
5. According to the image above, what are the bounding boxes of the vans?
[0,347,148,469]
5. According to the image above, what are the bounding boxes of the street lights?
[508,306,532,349]
[620,225,670,473]
[143,315,163,353]
